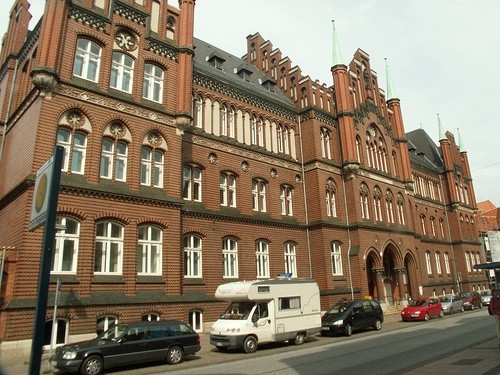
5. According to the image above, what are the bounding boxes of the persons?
[259,305,268,318]
[489,289,500,342]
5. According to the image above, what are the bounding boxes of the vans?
[210,278,324,352]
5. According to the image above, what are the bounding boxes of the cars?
[480,290,493,305]
[401,298,445,323]
[460,291,484,310]
[320,297,384,336]
[438,294,466,315]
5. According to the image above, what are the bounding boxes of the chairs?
[125,330,140,340]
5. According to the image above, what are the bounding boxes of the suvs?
[54,318,202,375]
